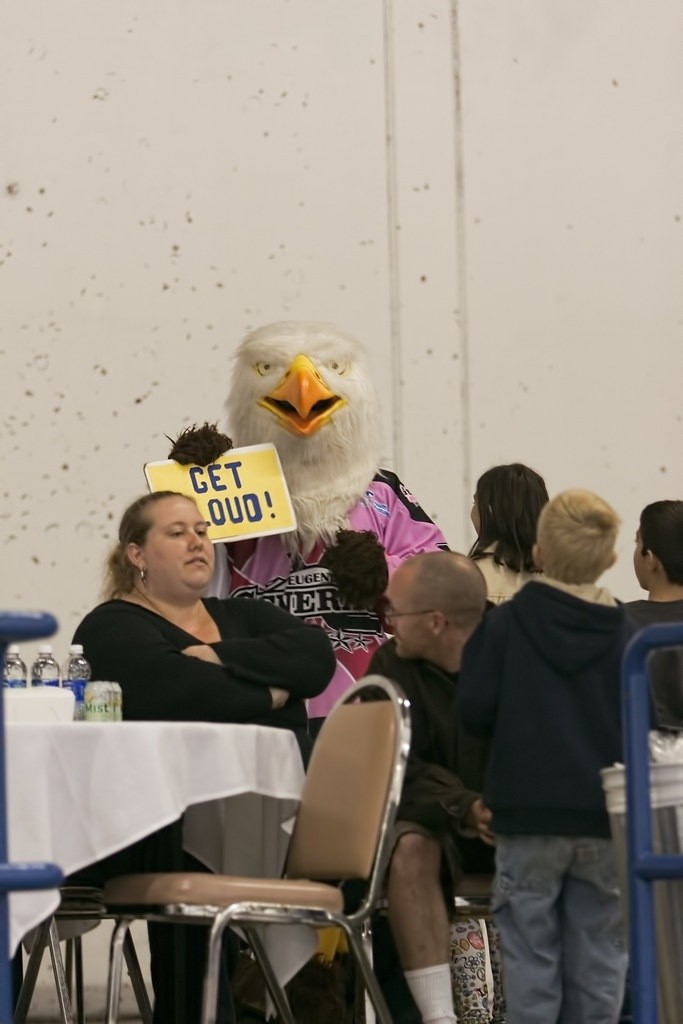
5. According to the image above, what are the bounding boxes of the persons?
[70,490,362,1024]
[362,461,683,1024]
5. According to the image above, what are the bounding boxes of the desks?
[4,721,305,963]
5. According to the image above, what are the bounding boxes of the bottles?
[1,644,92,721]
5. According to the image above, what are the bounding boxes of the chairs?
[14,673,497,1024]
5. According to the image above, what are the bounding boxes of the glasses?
[384,607,451,626]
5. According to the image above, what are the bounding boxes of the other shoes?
[287,955,348,1024]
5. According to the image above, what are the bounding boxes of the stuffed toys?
[168,319,453,774]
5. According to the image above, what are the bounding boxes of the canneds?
[84,681,122,722]
